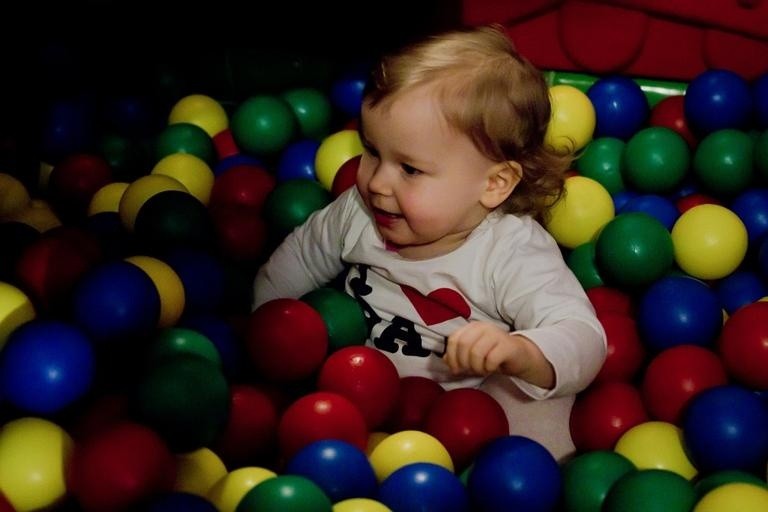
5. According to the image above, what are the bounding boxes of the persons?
[252,26,610,401]
